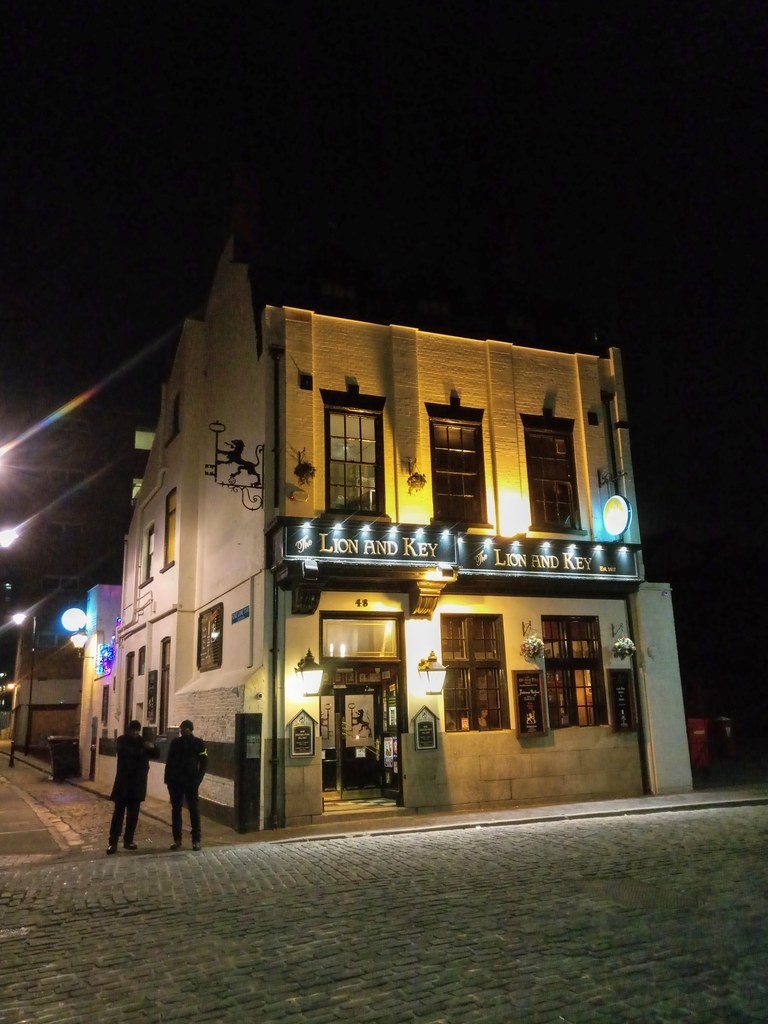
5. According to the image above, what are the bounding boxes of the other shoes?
[193,840,201,851]
[124,838,138,849]
[170,840,182,849]
[107,843,117,854]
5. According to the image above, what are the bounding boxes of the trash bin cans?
[48,736,82,780]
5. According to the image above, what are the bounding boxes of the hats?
[180,720,193,731]
[129,720,141,731]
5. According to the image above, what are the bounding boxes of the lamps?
[70,628,93,658]
[418,650,448,695]
[294,650,324,696]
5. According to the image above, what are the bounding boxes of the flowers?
[407,473,427,491]
[613,636,636,657]
[521,637,545,659]
[295,462,314,484]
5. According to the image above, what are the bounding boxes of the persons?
[106,720,161,855]
[164,720,208,851]
[476,706,489,727]
